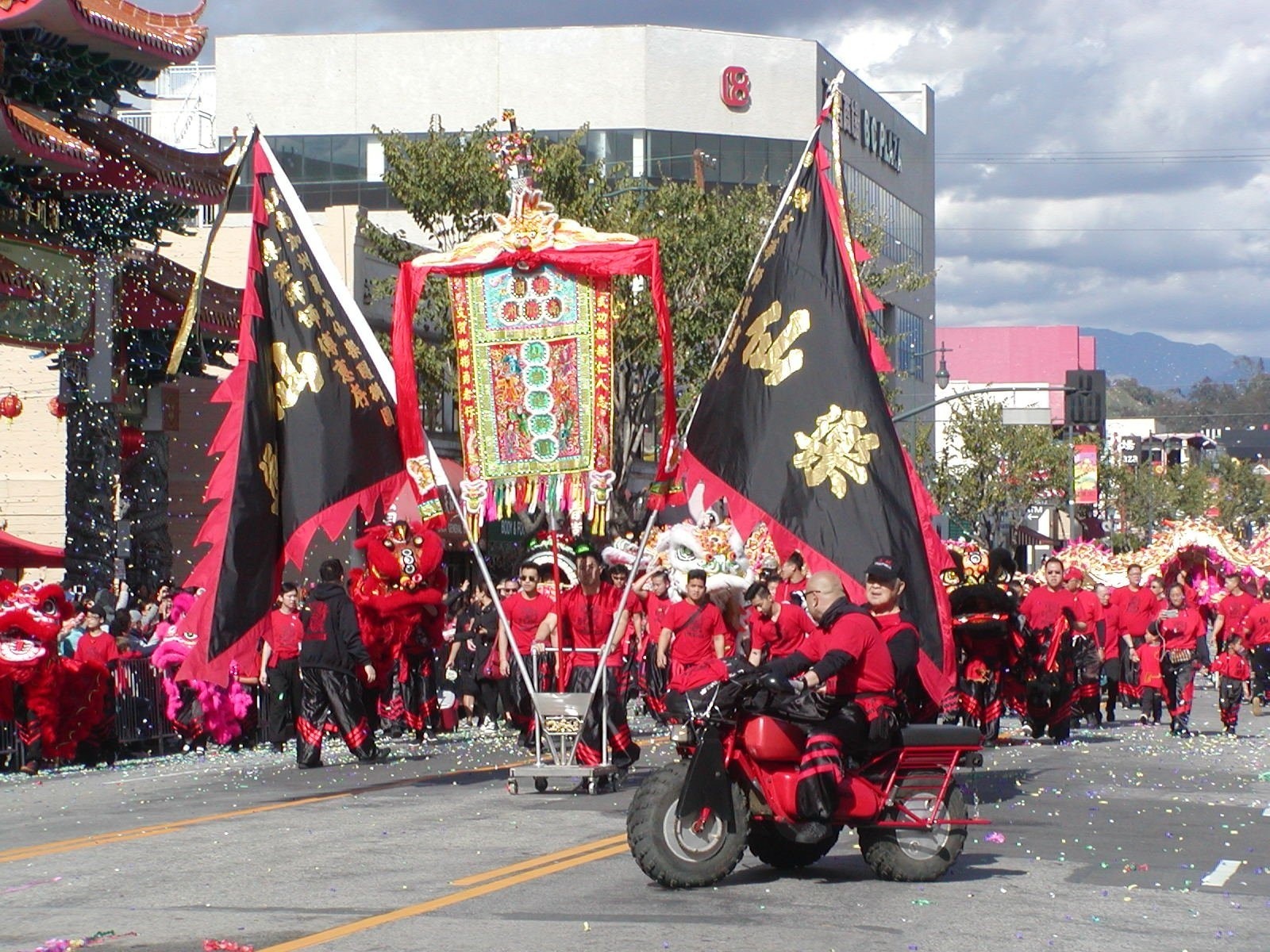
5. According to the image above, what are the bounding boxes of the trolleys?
[506,632,626,795]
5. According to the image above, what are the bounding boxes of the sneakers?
[795,821,834,844]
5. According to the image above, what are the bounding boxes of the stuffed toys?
[600,510,753,614]
[343,517,446,688]
[1,580,110,759]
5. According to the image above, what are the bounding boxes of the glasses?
[803,590,821,600]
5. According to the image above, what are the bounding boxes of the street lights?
[908,340,953,469]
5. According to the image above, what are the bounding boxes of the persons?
[294,558,391,769]
[941,548,1269,752]
[260,583,306,755]
[854,555,920,728]
[13,573,284,777]
[752,570,904,848]
[375,548,817,791]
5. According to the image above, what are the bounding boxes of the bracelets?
[795,676,809,692]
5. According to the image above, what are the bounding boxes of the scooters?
[620,635,988,878]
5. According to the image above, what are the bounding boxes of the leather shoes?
[360,748,391,764]
[299,762,323,768]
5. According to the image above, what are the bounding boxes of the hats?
[864,554,907,580]
